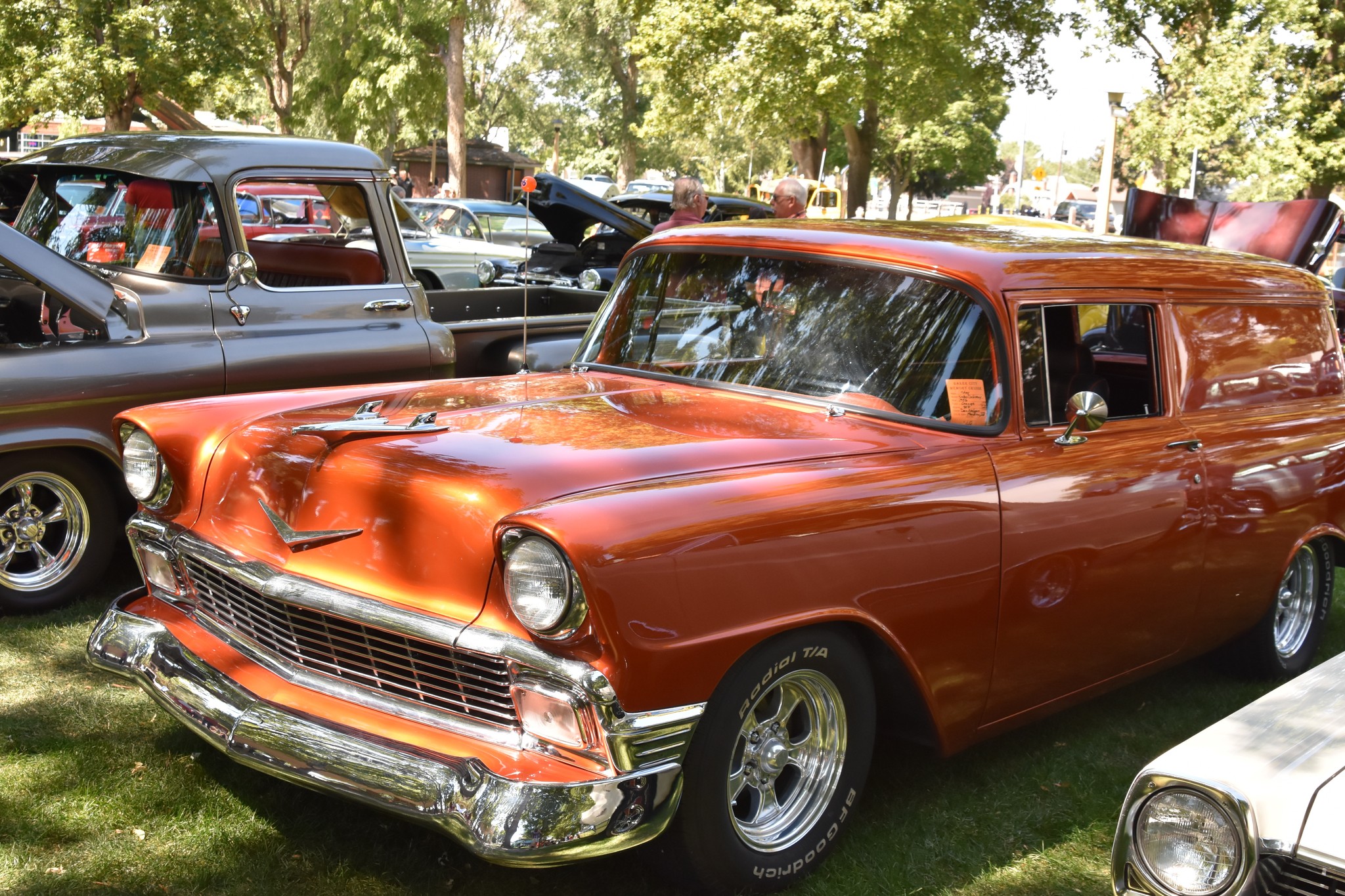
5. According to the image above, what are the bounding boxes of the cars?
[506,169,781,290]
[571,174,669,204]
[1108,649,1345,896]
[36,181,551,332]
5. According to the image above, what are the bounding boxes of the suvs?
[1051,200,1117,235]
[86,187,1344,896]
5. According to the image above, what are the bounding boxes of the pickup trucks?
[0,130,743,610]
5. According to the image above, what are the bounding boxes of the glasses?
[697,192,710,200]
[773,193,790,200]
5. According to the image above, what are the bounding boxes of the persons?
[124,177,175,261]
[651,175,727,302]
[426,181,456,199]
[388,167,415,199]
[756,178,808,311]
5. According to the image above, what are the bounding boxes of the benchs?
[195,238,384,287]
[485,233,556,246]
